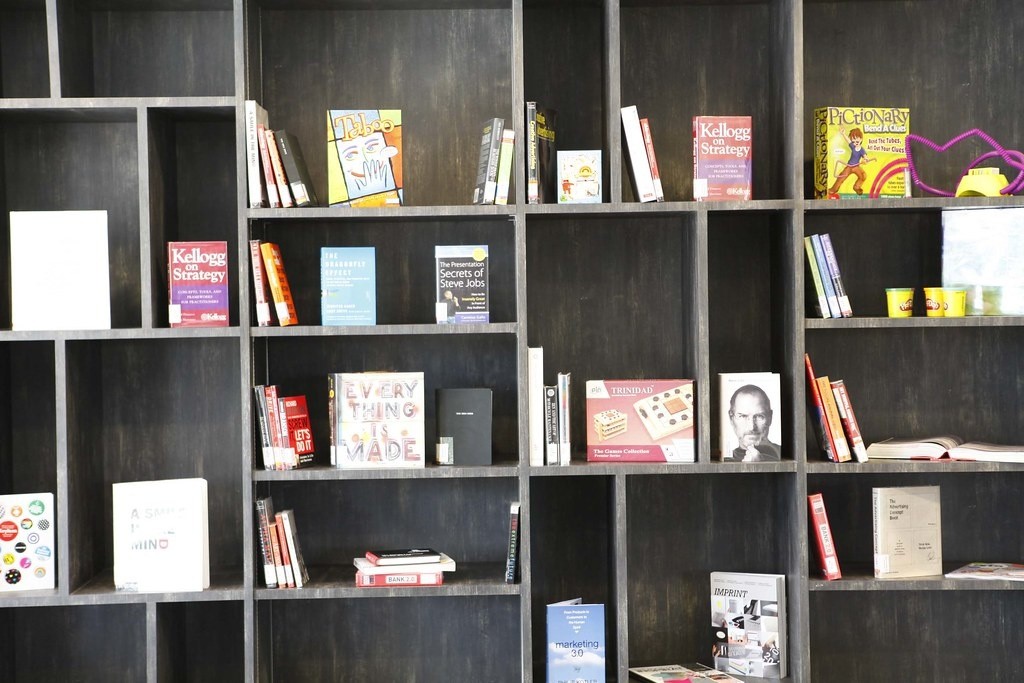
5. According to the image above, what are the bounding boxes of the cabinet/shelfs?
[0,0,1024,683]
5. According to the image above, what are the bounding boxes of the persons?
[724,385,781,461]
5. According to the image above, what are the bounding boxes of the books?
[528,345,570,467]
[807,485,1024,581]
[254,371,492,470]
[546,571,788,683]
[719,371,784,462]
[10,210,111,329]
[250,238,489,326]
[527,101,752,203]
[803,233,853,319]
[504,501,520,583]
[167,240,228,327]
[354,549,455,587]
[112,478,210,593]
[256,496,311,588]
[0,493,55,590]
[806,354,1024,463]
[244,100,514,208]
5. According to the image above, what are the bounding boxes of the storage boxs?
[813,107,910,200]
